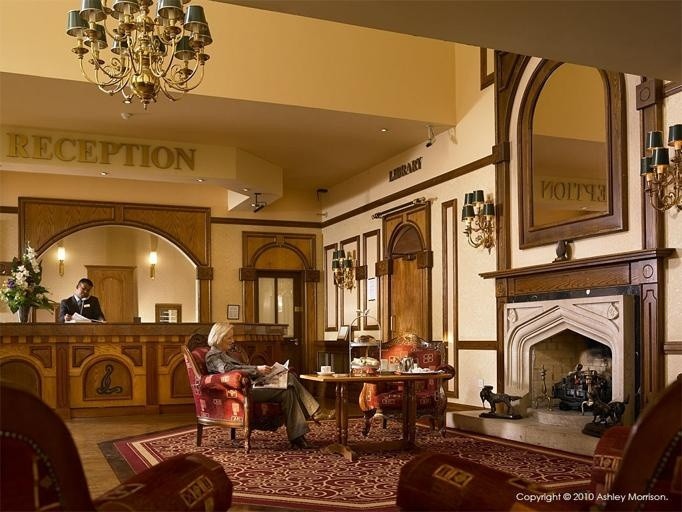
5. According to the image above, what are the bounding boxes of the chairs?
[359,334,456,437]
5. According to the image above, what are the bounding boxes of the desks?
[300,374,454,462]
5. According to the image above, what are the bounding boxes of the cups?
[320,365,332,372]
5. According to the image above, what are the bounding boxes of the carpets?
[97,416,593,511]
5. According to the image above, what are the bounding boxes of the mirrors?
[516,60,627,250]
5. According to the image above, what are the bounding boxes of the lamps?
[65,1,213,112]
[640,124,682,211]
[57,247,65,276]
[331,249,356,292]
[149,252,158,279]
[461,191,495,253]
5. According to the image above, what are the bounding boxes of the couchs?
[0,381,232,512]
[395,372,682,511]
[181,335,295,453]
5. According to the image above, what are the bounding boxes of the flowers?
[1,239,55,313]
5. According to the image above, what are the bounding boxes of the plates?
[400,370,443,375]
[316,371,335,376]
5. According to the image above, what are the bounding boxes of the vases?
[18,307,30,321]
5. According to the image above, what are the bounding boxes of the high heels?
[313,408,336,426]
[291,435,319,449]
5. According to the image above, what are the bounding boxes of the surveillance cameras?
[425,136,437,148]
[253,201,265,212]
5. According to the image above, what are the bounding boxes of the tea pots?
[399,356,414,372]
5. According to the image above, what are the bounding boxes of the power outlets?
[478,378,484,388]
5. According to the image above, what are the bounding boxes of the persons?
[58,277,106,325]
[203,317,338,452]
